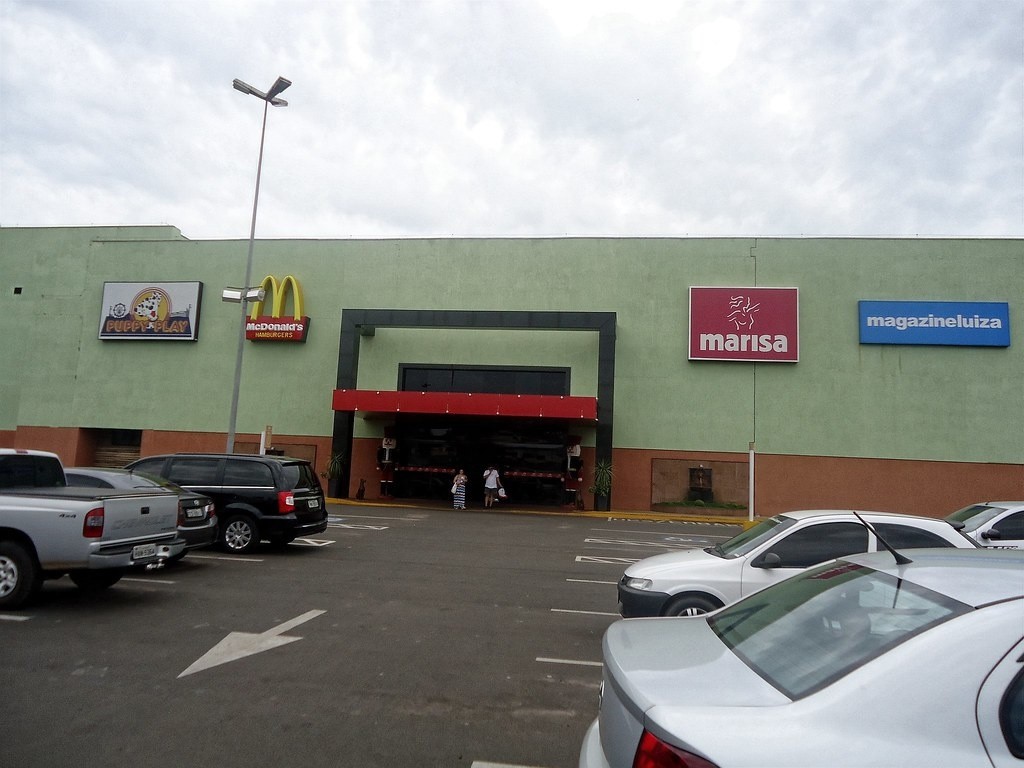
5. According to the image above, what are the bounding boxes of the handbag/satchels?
[450,482,457,494]
[498,488,506,497]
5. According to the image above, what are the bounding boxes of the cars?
[62,465,219,569]
[568,544,1024,768]
[612,508,988,624]
[935,499,1024,552]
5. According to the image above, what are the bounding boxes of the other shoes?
[461,507,466,510]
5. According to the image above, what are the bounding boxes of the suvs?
[119,452,330,557]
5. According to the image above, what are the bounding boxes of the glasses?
[490,468,494,469]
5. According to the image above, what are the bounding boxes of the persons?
[482,466,504,509]
[453,469,468,509]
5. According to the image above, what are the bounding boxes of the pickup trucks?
[0,447,188,609]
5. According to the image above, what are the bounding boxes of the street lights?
[220,77,297,458]
